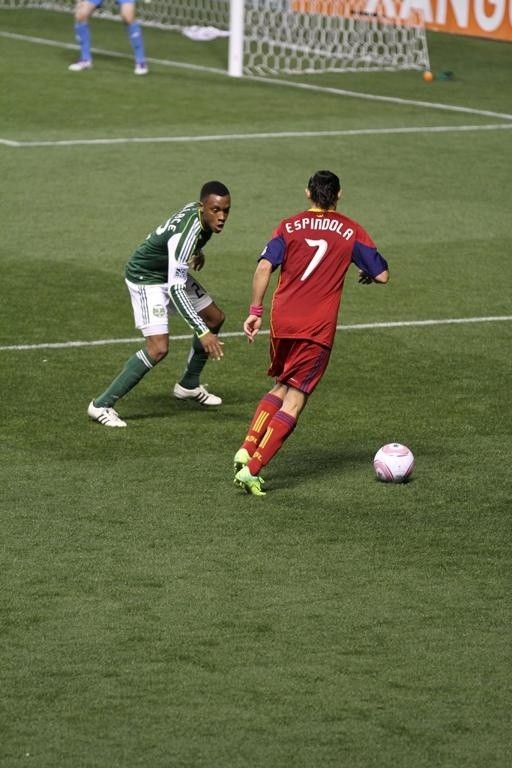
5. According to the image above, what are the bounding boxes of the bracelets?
[249,305,264,317]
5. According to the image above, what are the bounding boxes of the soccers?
[373,443,414,483]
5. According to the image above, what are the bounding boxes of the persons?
[87,181,231,427]
[234,170,389,496]
[69,0,148,76]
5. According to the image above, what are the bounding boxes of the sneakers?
[173,382,222,406]
[68,61,93,71]
[133,63,148,75]
[87,399,127,427]
[234,448,266,496]
